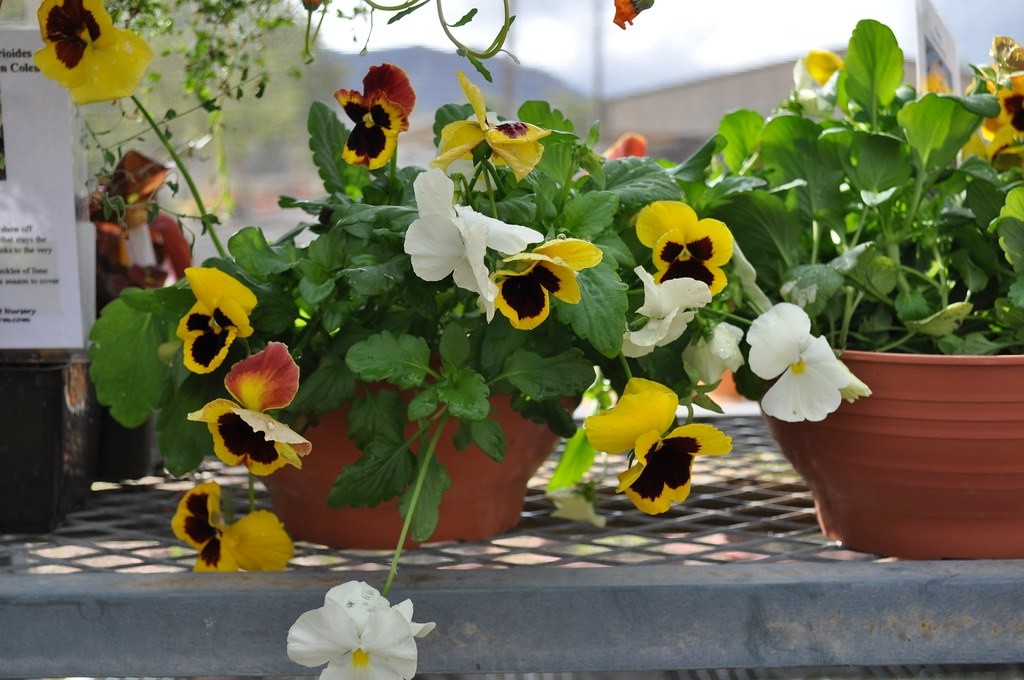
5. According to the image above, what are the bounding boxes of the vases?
[757,352,1024,561]
[259,354,576,549]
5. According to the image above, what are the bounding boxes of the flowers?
[33,0,1024,680]
